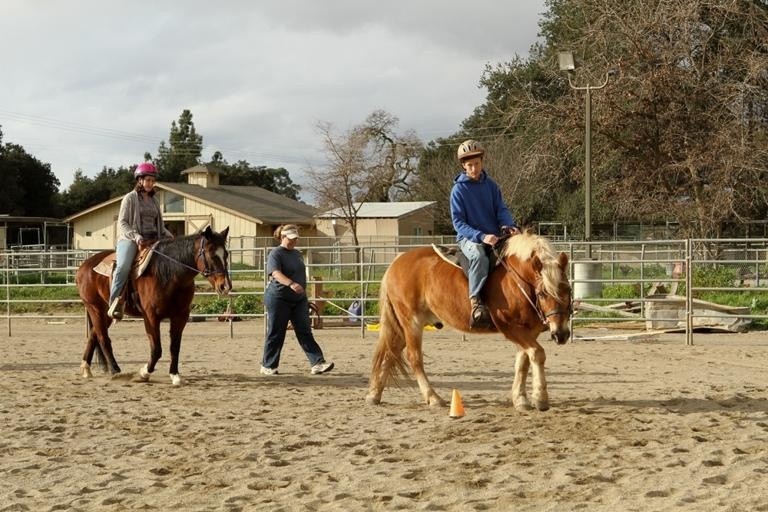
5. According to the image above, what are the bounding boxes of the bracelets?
[288,280,295,286]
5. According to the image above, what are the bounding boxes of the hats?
[281,229,299,239]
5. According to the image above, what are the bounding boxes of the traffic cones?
[445,386,469,421]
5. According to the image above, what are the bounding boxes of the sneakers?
[261,366,278,374]
[311,362,334,375]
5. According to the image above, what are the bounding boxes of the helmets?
[135,163,159,180]
[458,140,483,159]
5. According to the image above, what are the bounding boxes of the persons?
[259,224,335,377]
[450,139,521,328]
[106,162,174,318]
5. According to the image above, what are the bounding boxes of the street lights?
[556,49,618,240]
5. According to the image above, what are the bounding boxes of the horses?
[364,227,573,412]
[75,225,232,388]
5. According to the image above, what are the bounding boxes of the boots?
[471,297,486,319]
